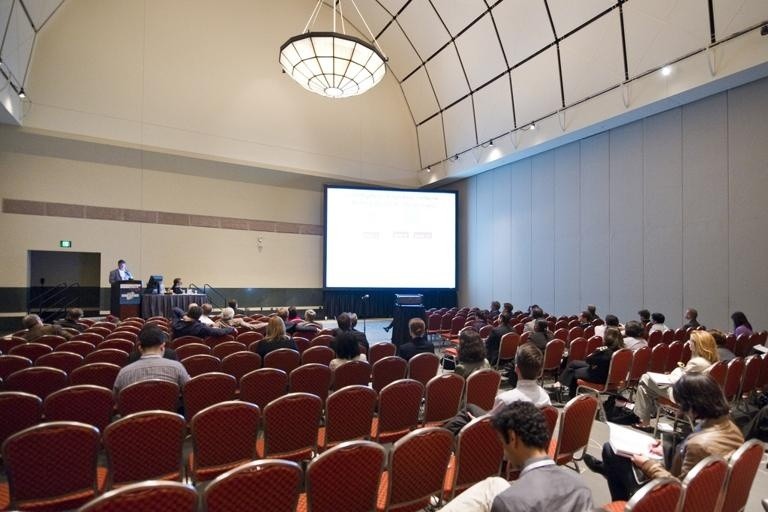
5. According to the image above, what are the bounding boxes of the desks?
[141,293,207,320]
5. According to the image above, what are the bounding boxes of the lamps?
[278,0,387,101]
[424,122,536,173]
[18,87,26,98]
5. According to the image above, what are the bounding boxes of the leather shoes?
[582,453,606,479]
[543,384,563,392]
[631,423,653,434]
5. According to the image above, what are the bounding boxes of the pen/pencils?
[649,441,661,453]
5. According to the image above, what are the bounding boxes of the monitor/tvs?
[147,275,163,294]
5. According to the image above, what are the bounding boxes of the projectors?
[394,293,422,304]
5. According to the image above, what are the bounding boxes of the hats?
[223,307,234,319]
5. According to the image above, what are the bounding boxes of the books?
[606,421,664,459]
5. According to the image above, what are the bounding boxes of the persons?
[396,318,435,361]
[111,325,192,394]
[383,319,394,332]
[128,322,178,362]
[583,371,746,502]
[53,307,85,332]
[437,399,594,512]
[109,260,133,285]
[172,298,370,368]
[19,313,73,343]
[436,300,768,442]
[172,278,184,294]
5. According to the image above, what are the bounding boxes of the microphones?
[361,293,370,300]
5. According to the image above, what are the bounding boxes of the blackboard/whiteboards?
[26,250,101,311]
